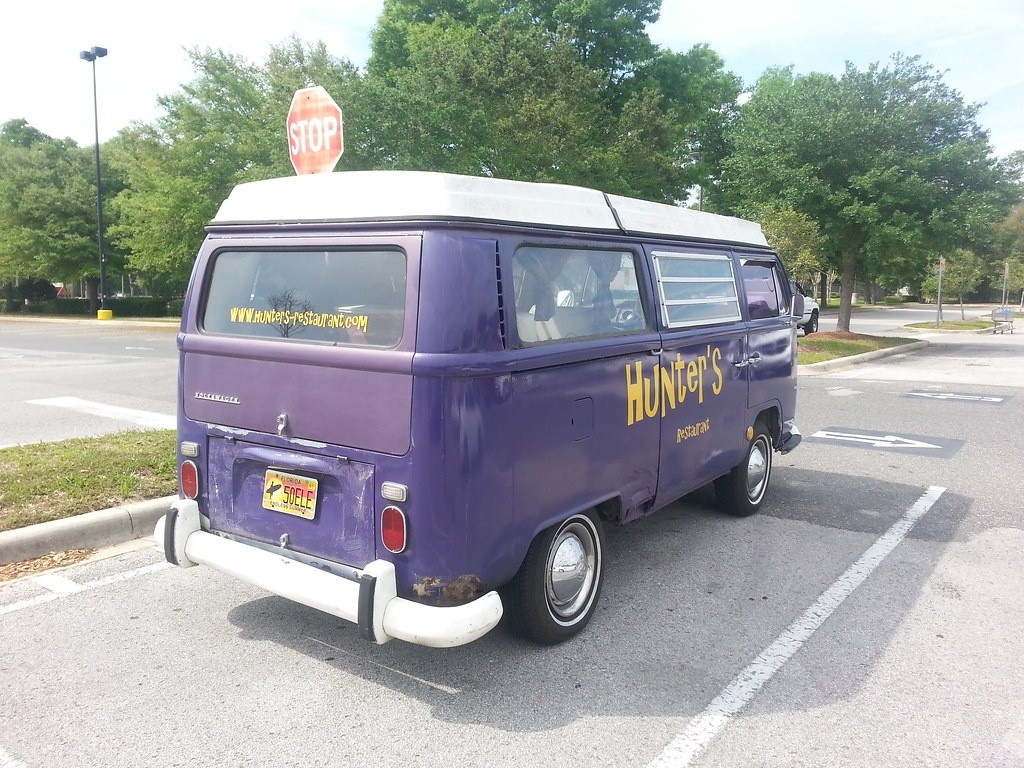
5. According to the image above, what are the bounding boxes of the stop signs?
[284,85,345,174]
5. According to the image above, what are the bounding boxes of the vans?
[151,170,801,652]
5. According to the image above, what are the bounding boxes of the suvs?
[788,279,820,334]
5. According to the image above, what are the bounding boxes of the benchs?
[336,306,595,346]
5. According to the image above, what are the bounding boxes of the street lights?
[79,44,116,320]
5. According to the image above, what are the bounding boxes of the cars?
[111,291,126,298]
[97,293,106,300]
